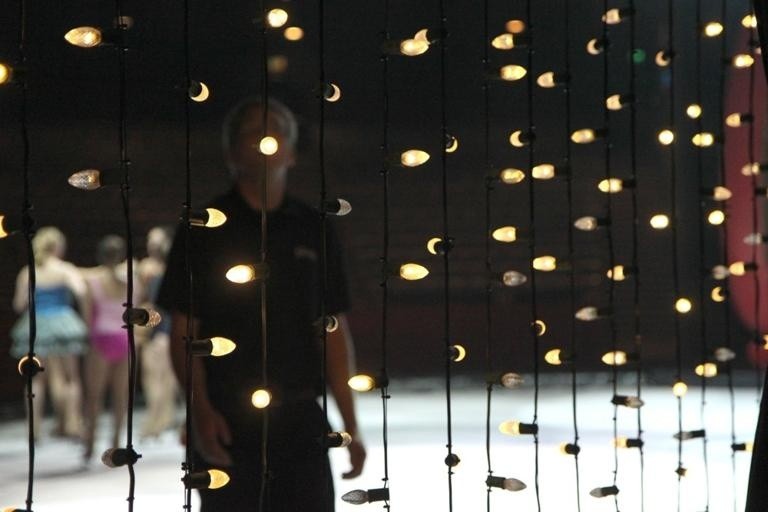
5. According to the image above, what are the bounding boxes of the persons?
[79,235,151,464]
[153,89,368,512]
[10,228,95,446]
[132,226,185,441]
